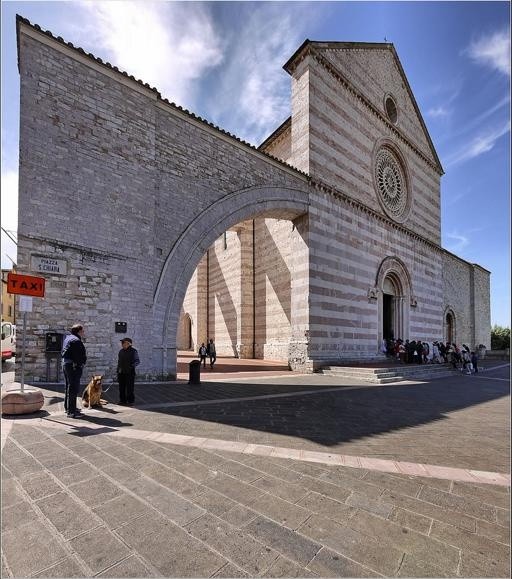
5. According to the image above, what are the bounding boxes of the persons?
[207,338,216,369]
[118,337,141,405]
[60,323,87,418]
[198,342,207,368]
[382,337,478,375]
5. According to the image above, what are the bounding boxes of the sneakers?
[64,408,84,418]
[199,362,215,370]
[115,400,135,408]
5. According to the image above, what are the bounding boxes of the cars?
[0,322,15,361]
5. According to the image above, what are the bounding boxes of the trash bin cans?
[188,360,201,384]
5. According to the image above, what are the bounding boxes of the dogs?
[81,375,108,409]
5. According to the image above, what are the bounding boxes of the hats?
[120,338,132,344]
